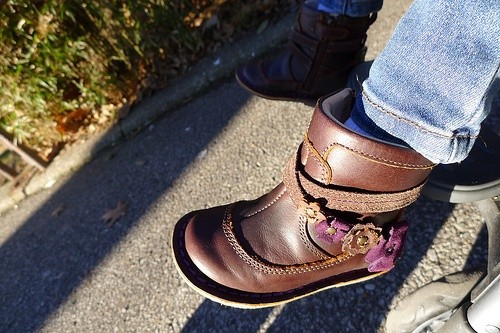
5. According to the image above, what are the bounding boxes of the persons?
[171,0,497,309]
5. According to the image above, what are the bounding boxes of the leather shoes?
[170,86,441,310]
[235,9,378,101]
